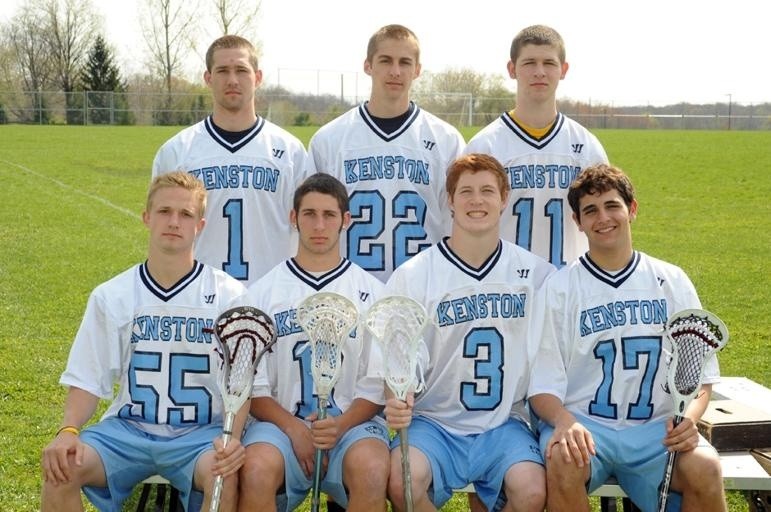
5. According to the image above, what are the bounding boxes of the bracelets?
[54,425,79,436]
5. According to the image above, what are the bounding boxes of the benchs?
[130,425,771,511]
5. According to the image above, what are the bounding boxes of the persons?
[307,23,466,293]
[526,162,733,512]
[230,171,391,511]
[39,169,254,512]
[149,34,320,292]
[367,150,549,512]
[457,24,605,274]
